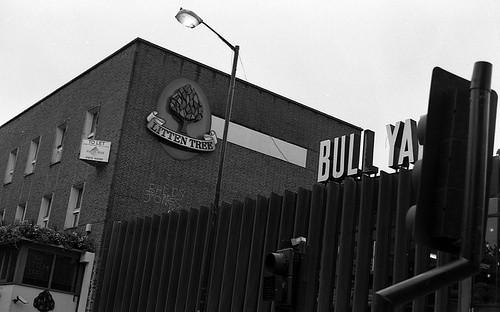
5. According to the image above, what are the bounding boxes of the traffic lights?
[265,248,293,307]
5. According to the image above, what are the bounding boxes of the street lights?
[174,7,240,312]
